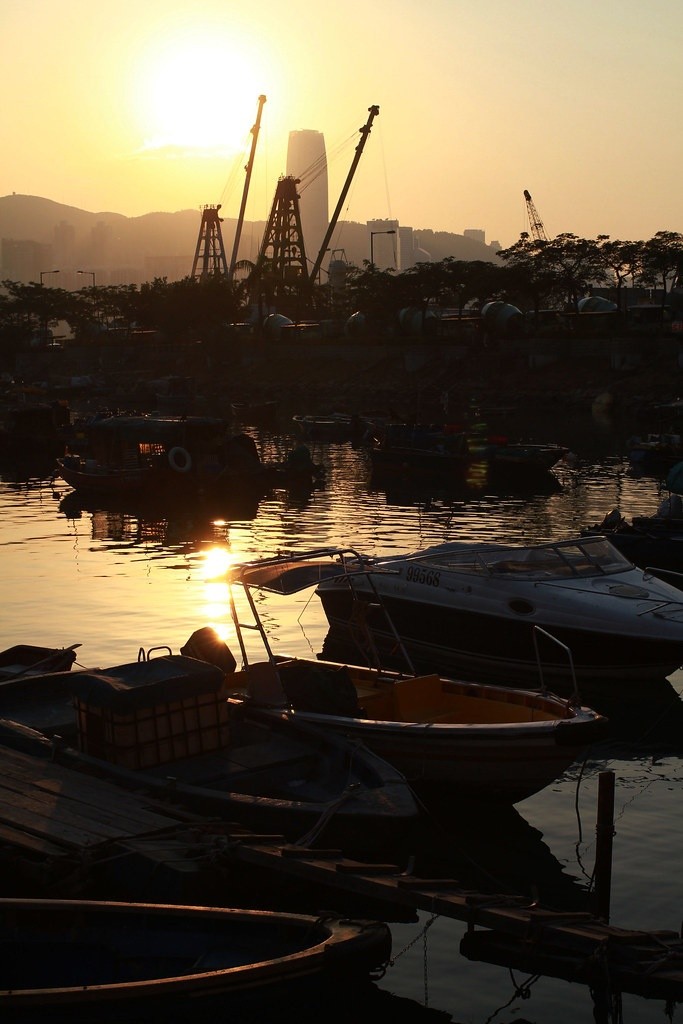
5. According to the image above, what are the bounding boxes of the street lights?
[371,231,395,262]
[40,270,59,284]
[79,271,95,316]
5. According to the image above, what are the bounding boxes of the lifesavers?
[167,446,193,473]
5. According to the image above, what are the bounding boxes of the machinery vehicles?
[229,95,379,285]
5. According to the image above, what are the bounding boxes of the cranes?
[192,176,552,280]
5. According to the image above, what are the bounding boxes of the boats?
[55,417,234,494]
[0,645,75,682]
[292,414,352,437]
[262,446,324,501]
[0,630,233,758]
[362,424,568,486]
[316,536,683,685]
[230,401,279,422]
[222,550,607,813]
[155,377,220,415]
[1,896,391,1024]
[318,626,683,762]
[629,402,682,475]
[345,409,411,438]
[0,386,69,454]
